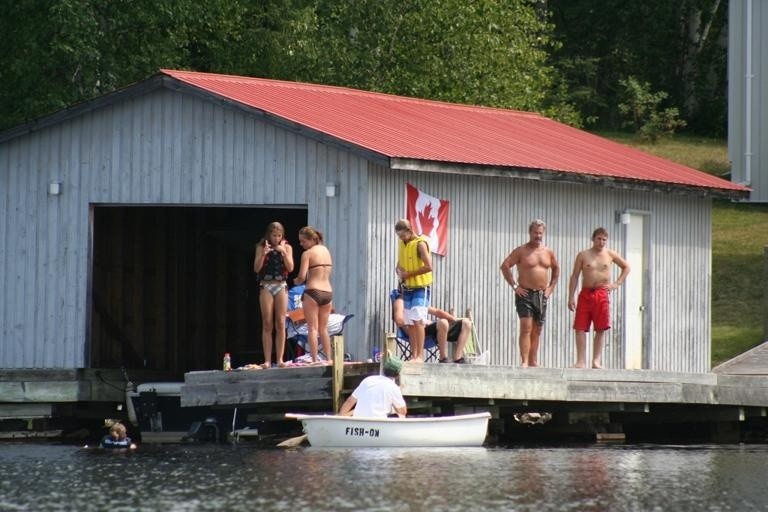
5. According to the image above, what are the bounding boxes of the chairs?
[394,325,440,364]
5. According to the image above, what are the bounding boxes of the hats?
[380,355,403,375]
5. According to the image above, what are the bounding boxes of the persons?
[394,281,471,363]
[568,228,631,369]
[339,356,406,418]
[294,226,333,363]
[253,222,293,369]
[395,219,433,363]
[501,219,560,369]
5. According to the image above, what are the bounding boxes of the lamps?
[325,183,339,197]
[614,210,631,225]
[48,181,61,196]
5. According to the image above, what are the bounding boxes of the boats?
[282,413,492,447]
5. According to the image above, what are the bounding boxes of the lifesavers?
[102,435,132,449]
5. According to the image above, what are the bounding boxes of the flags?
[405,182,449,257]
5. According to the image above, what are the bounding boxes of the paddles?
[275,409,354,447]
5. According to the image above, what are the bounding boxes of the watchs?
[513,284,518,289]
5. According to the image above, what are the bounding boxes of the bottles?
[223,352,231,371]
